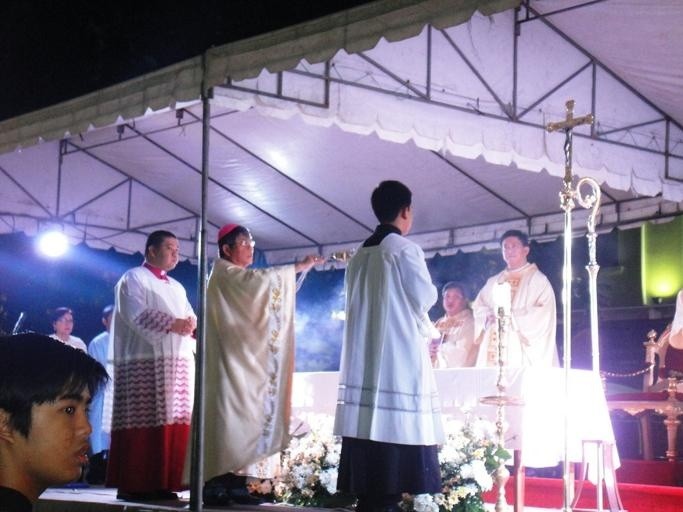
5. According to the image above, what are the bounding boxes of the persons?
[471,230,561,370]
[431,281,480,367]
[47,305,89,354]
[202,222,325,507]
[105,231,198,504]
[333,180,440,511]
[654,290,683,462]
[86,304,115,486]
[0,333,111,511]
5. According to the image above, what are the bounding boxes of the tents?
[0,1,683,508]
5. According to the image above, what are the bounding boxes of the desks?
[237,366,622,511]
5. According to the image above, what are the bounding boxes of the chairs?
[592,321,683,465]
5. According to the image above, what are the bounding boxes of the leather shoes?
[202,487,234,508]
[231,491,264,504]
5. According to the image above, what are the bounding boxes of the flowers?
[246,429,511,511]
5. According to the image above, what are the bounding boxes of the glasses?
[235,238,256,248]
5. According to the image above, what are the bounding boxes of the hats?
[218,223,239,243]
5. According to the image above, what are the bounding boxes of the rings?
[314,257,318,261]
